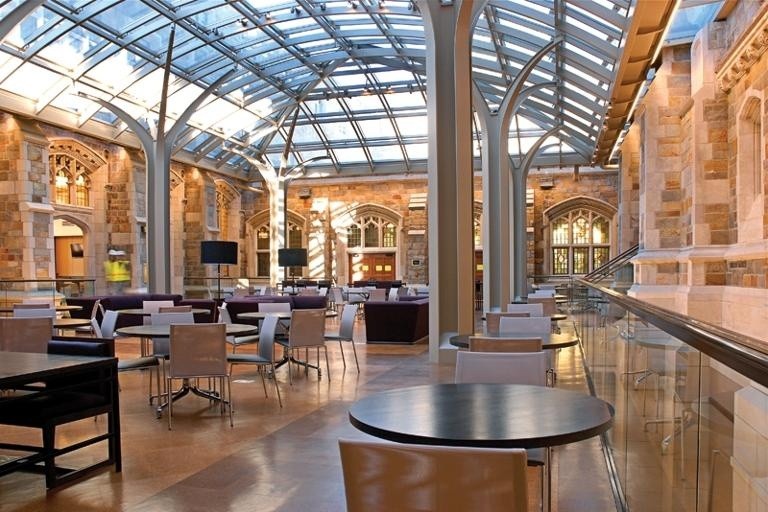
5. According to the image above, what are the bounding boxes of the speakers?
[278,248,308,267]
[200,240,238,265]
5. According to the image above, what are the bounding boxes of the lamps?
[211,27,218,35]
[278,248,307,296]
[201,241,237,300]
[320,3,326,11]
[290,6,299,14]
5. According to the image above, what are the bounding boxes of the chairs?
[455,352,552,512]
[450,285,578,351]
[340,440,529,512]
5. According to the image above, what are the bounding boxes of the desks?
[578,295,733,456]
[349,382,615,450]
